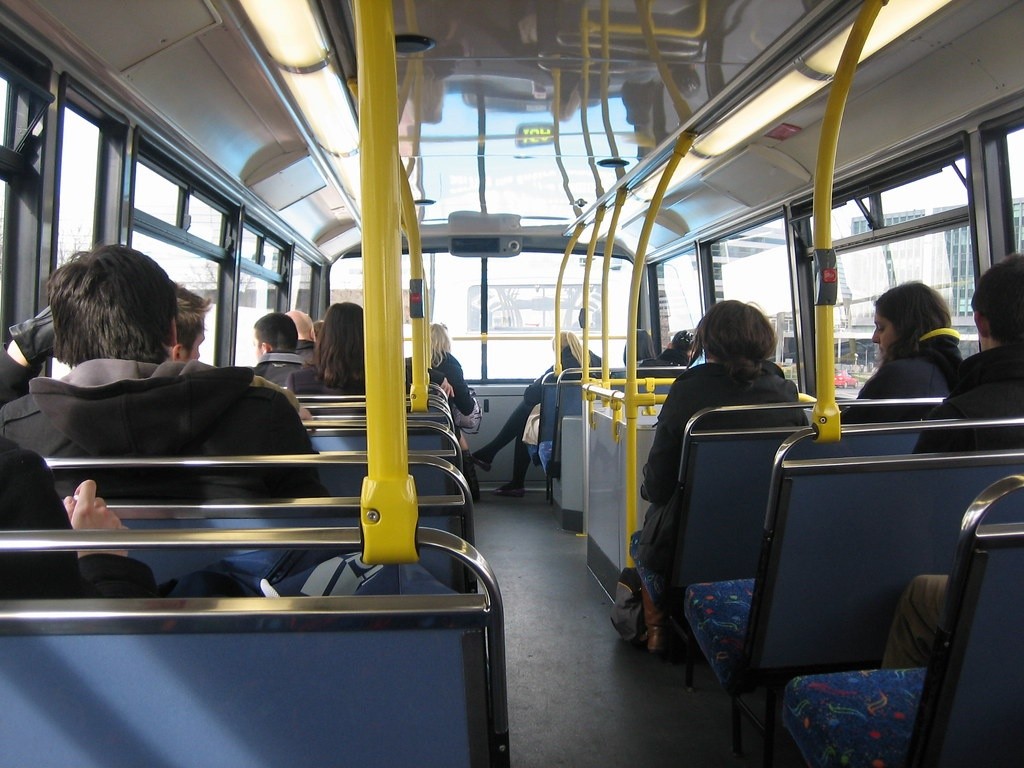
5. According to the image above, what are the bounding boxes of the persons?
[882,574,948,669]
[471,331,601,498]
[630,299,810,654]
[429,322,481,501]
[252,302,454,398]
[839,282,962,422]
[911,253,1024,454]
[0,437,177,600]
[622,331,695,378]
[0,243,328,499]
[175,286,317,421]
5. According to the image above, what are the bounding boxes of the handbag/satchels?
[610,567,685,644]
[449,388,482,435]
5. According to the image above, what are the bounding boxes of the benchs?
[629,397,947,690]
[0,385,519,768]
[527,370,578,500]
[538,366,693,505]
[763,474,1024,768]
[681,416,1024,754]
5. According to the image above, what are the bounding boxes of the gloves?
[9,305,56,367]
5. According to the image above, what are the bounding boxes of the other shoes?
[492,484,525,497]
[472,453,492,472]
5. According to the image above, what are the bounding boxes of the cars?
[834,370,858,388]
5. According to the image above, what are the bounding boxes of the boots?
[461,450,480,500]
[640,580,666,653]
